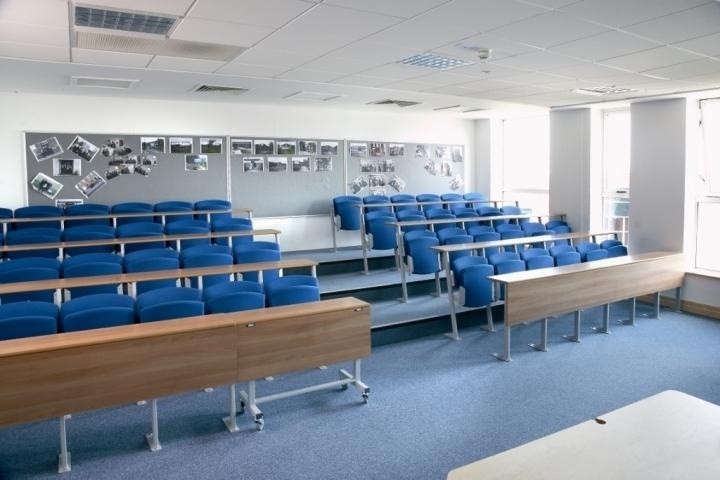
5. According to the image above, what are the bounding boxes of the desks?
[447,389,720,480]
[492,252,685,363]
